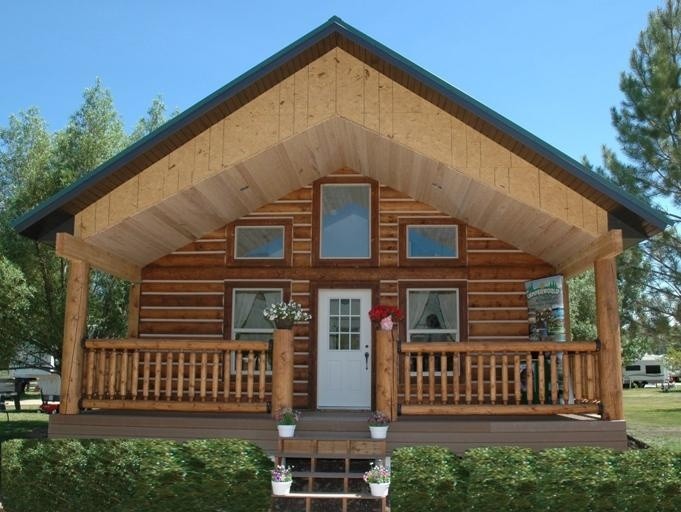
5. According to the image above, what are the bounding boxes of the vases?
[274,318,293,329]
[380,315,393,331]
[276,424,296,438]
[368,482,390,496]
[271,480,292,496]
[369,425,388,439]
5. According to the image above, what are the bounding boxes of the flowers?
[366,409,390,427]
[273,404,304,425]
[361,459,390,484]
[269,464,295,482]
[263,298,312,321]
[368,304,405,322]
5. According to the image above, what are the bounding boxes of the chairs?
[0,378,20,411]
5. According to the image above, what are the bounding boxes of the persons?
[422,313,450,346]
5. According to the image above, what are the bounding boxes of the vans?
[620,356,666,389]
